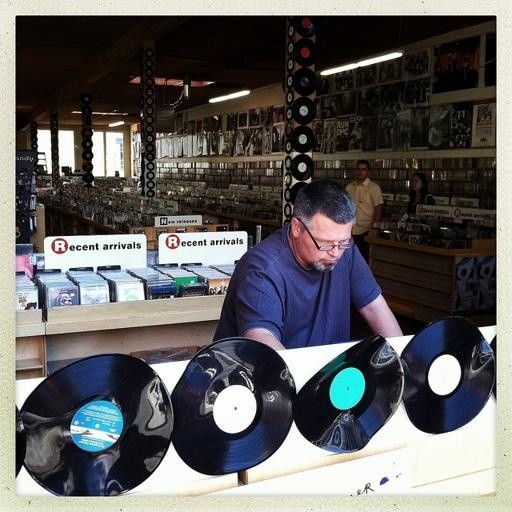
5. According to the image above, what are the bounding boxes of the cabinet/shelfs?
[39,197,282,251]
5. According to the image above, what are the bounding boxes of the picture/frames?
[154,21,496,162]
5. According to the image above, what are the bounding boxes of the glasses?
[296,217,355,251]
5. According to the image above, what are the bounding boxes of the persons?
[398,118,413,152]
[342,158,385,264]
[209,285,227,296]
[347,121,364,151]
[209,178,404,351]
[405,172,436,218]
